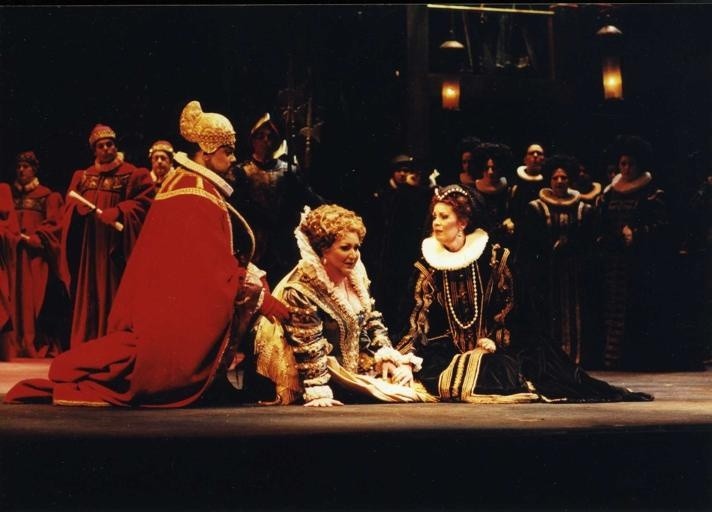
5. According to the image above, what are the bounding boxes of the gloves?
[258,288,290,324]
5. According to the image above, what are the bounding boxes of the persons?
[1,98,711,408]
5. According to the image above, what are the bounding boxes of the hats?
[148,141,173,159]
[179,100,236,154]
[90,124,116,144]
[16,151,39,173]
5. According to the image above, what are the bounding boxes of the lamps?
[433,24,468,113]
[593,5,626,104]
